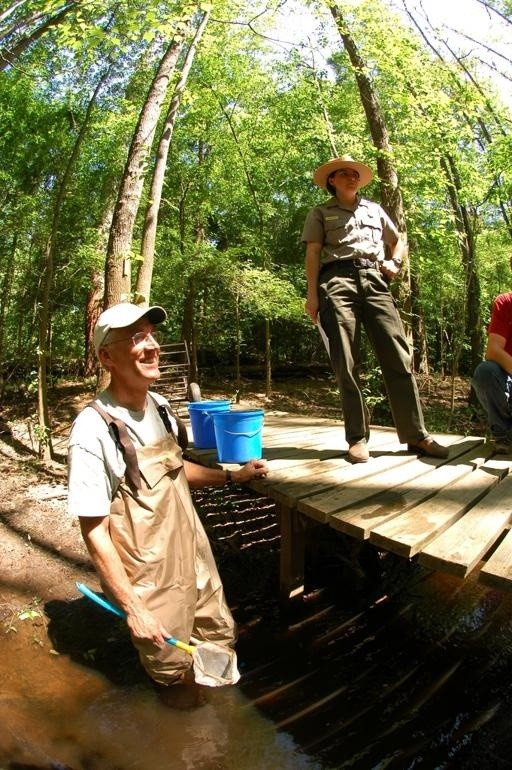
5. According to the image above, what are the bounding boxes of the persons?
[68,303,268,686]
[471,255,512,454]
[304,158,449,464]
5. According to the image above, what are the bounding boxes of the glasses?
[104,331,164,349]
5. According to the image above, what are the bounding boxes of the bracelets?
[226,471,232,486]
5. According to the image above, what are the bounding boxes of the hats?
[313,154,373,189]
[94,303,167,356]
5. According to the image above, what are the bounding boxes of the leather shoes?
[408,434,449,458]
[348,437,369,462]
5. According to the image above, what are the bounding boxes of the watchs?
[392,257,403,268]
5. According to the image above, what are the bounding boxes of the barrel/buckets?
[212,409,266,463]
[188,400,232,449]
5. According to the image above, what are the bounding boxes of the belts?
[322,256,383,273]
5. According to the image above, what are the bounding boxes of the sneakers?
[492,435,512,454]
